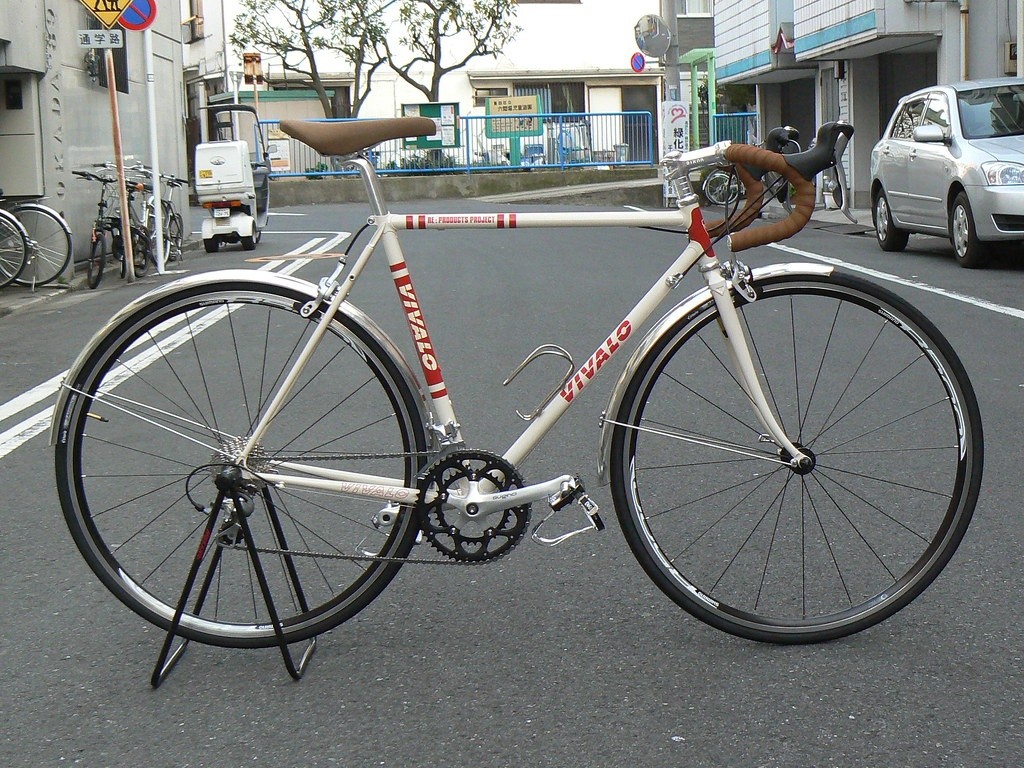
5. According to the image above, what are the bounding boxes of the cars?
[868,76,1024,267]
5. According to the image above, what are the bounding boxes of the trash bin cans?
[614,143,630,166]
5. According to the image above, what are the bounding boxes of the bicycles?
[0,188,73,292]
[47,119,985,685]
[702,139,787,205]
[67,160,192,288]
